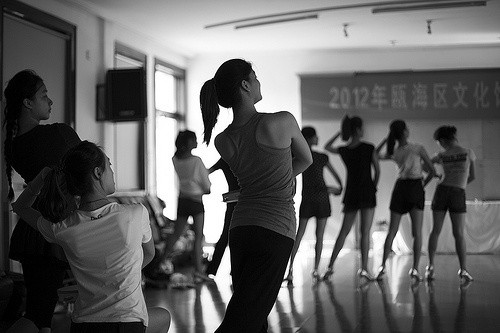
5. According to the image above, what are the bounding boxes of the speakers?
[106,69,148,120]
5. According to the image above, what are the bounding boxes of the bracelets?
[26,181,40,198]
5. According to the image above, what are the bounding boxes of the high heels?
[409,268,424,281]
[312,271,321,282]
[425,265,435,280]
[322,270,334,281]
[376,266,385,281]
[193,271,210,283]
[287,274,293,286]
[357,269,375,281]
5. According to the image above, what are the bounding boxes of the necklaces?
[80,197,107,206]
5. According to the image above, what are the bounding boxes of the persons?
[199,58,313,333]
[158,130,210,281]
[421,125,477,283]
[13,141,155,333]
[323,115,381,286]
[0,270,39,333]
[3,69,82,333]
[286,126,343,283]
[203,157,241,283]
[376,120,436,281]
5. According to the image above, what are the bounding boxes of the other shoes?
[458,268,474,282]
